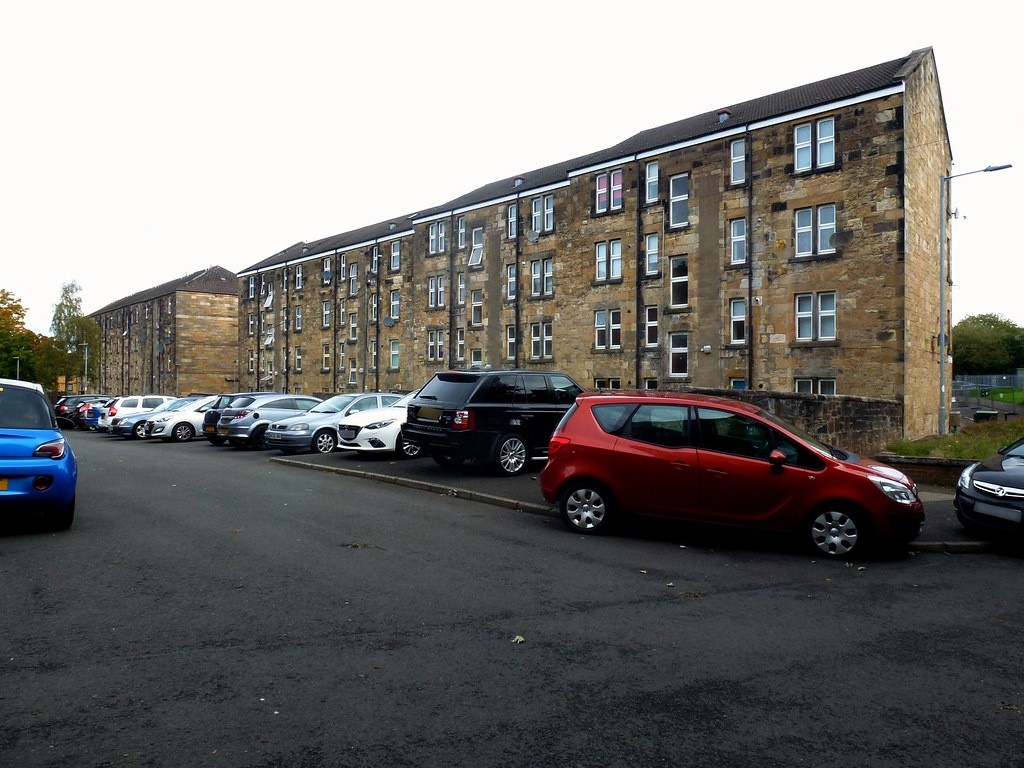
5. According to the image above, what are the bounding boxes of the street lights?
[939,163,1012,436]
[78,343,88,394]
[13,357,20,380]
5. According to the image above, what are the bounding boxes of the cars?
[144,396,218,443]
[400,369,586,475]
[0,379,78,533]
[337,389,423,460]
[264,393,404,454]
[54,395,116,427]
[109,397,200,440]
[954,437,1024,544]
[216,394,341,450]
[73,399,111,431]
[98,395,189,433]
[200,392,282,446]
[540,390,925,562]
